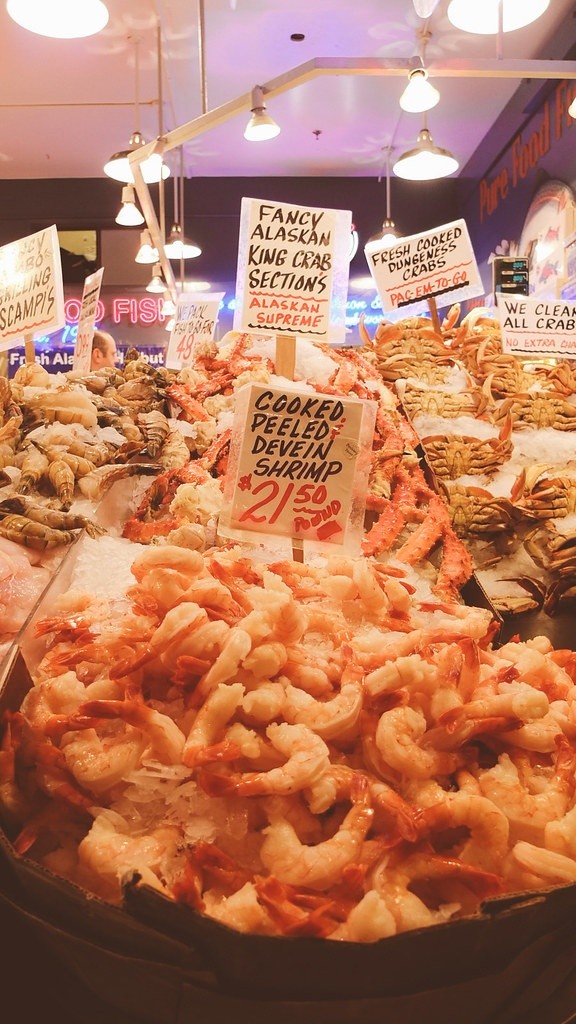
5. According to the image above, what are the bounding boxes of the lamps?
[115,183,144,226]
[391,109,461,182]
[145,262,167,294]
[365,144,407,247]
[160,292,178,316]
[163,145,203,259]
[135,228,160,264]
[399,56,441,114]
[242,84,282,144]
[101,33,171,183]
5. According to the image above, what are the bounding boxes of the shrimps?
[1,357,176,557]
[1,523,576,943]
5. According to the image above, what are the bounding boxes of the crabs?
[358,302,576,621]
[120,331,476,597]
